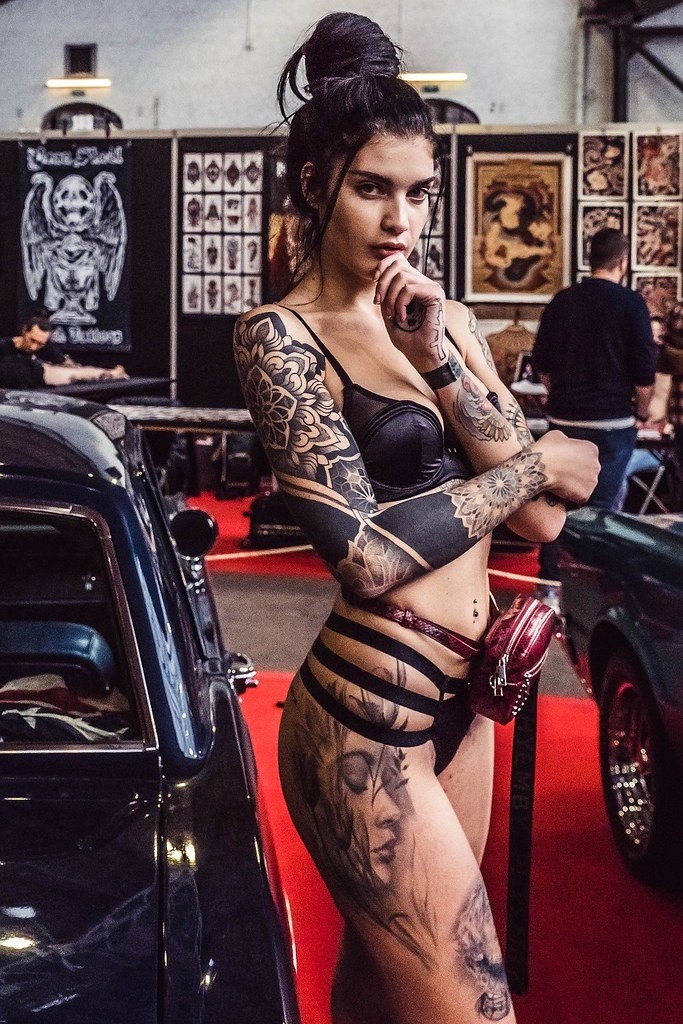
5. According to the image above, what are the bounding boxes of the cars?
[538,507,682,885]
[1,390,299,1024]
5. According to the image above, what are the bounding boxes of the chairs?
[0,621,118,700]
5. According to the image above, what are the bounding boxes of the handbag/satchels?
[341,581,556,725]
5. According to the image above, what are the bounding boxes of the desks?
[108,403,255,498]
[522,418,670,516]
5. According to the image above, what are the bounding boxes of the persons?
[232,9,601,1024]
[0,356,129,388]
[530,227,656,512]
[609,345,674,512]
[0,310,74,367]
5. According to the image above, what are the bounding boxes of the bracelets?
[634,411,649,422]
[420,354,462,391]
